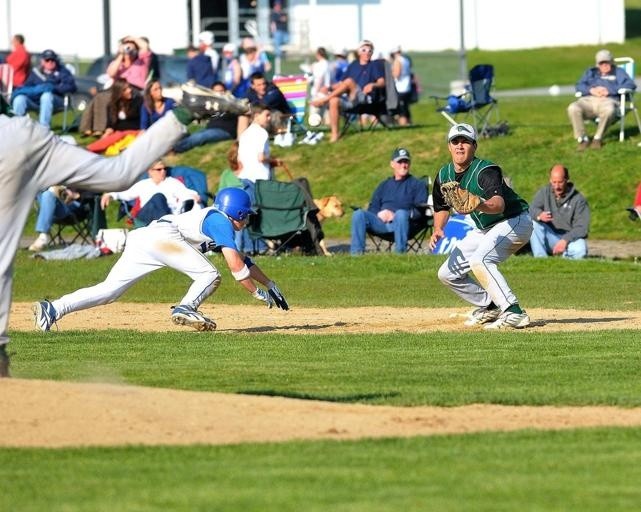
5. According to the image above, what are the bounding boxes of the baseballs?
[308,113,321,126]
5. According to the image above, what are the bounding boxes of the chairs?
[118,164,216,229]
[47,195,96,244]
[339,60,387,138]
[365,175,434,253]
[575,57,641,142]
[430,64,498,139]
[247,178,324,255]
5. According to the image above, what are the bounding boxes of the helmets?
[214,187,258,221]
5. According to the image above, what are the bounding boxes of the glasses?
[151,167,167,171]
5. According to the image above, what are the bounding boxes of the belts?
[157,220,172,223]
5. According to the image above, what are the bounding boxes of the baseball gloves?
[440,181,483,214]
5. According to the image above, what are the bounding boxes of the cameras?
[123,45,130,51]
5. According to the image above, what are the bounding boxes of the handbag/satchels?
[394,76,411,92]
[97,227,128,255]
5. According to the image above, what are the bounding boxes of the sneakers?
[576,139,603,152]
[465,308,502,327]
[0,348,10,378]
[182,84,248,120]
[486,312,530,329]
[28,232,54,251]
[171,305,216,331]
[32,301,56,333]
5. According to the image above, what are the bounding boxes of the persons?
[526,164,591,259]
[2,31,293,257]
[310,39,419,143]
[0,83,248,378]
[270,0,290,57]
[428,123,535,331]
[350,147,427,257]
[565,48,637,152]
[32,187,288,332]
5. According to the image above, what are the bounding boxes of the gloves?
[251,288,274,308]
[266,280,289,310]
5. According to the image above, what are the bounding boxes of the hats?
[358,40,373,48]
[42,50,57,60]
[596,49,612,63]
[448,123,476,142]
[393,148,411,162]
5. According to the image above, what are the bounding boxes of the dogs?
[313,195,346,258]
